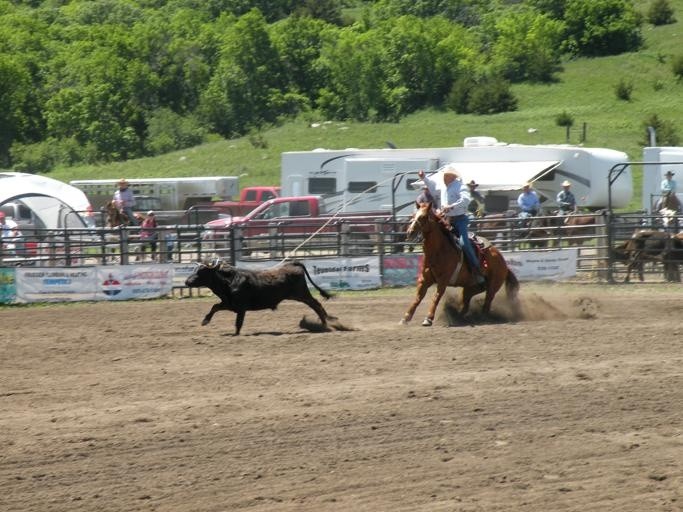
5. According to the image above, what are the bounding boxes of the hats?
[147,211,155,214]
[521,181,533,190]
[561,179,573,187]
[0,211,7,218]
[443,166,460,178]
[466,180,479,187]
[422,184,430,190]
[663,170,676,177]
[116,178,129,184]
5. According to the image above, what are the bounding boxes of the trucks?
[71,176,237,222]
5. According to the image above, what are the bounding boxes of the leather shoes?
[475,274,486,285]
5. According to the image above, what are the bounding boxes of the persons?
[0,211,24,268]
[556,179,576,223]
[418,167,481,272]
[656,170,680,213]
[517,181,539,228]
[112,179,139,226]
[135,210,157,260]
[415,186,437,214]
[466,179,485,218]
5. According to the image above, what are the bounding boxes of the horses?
[398,200,520,326]
[546,210,595,269]
[510,210,558,252]
[468,214,514,246]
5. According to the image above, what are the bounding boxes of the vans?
[0,202,33,231]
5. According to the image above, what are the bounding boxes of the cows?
[662,231,683,282]
[613,230,675,282]
[185,260,334,335]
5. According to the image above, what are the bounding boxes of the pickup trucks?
[201,195,391,259]
[92,195,218,242]
[207,186,281,217]
